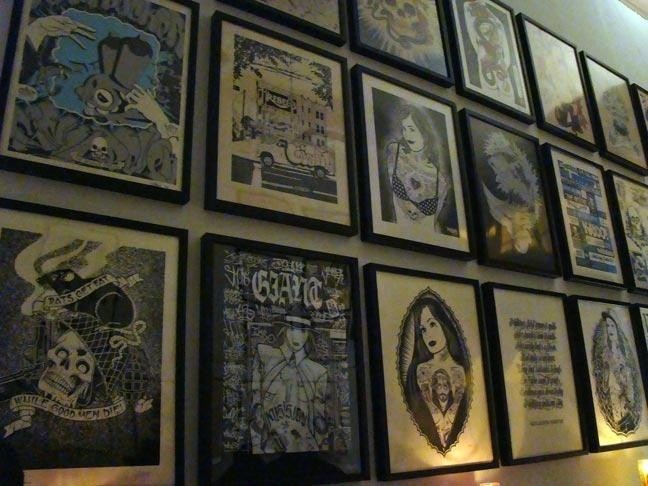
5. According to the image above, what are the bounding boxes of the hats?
[274,304,321,334]
[602,308,619,328]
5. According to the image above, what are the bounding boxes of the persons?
[409,299,468,446]
[602,311,635,430]
[251,304,329,451]
[387,105,450,230]
